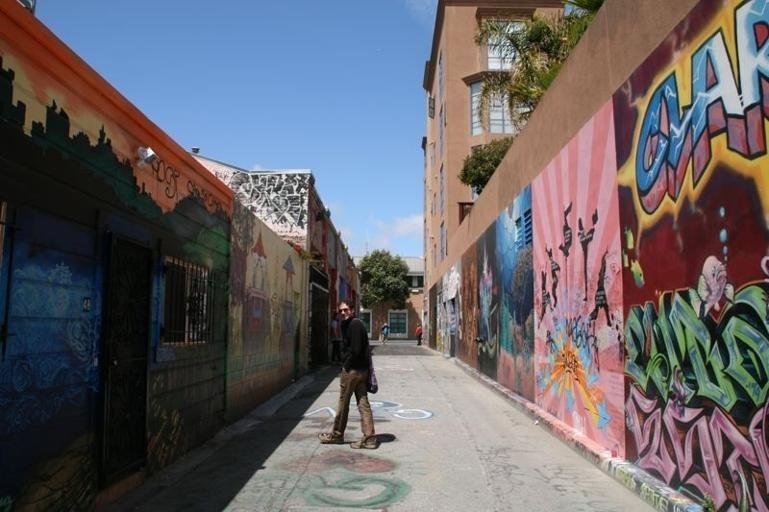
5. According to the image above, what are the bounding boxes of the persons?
[315,299,380,450]
[329,311,343,366]
[374,313,389,344]
[414,322,422,346]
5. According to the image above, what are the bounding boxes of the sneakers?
[350,441,377,449]
[318,433,344,444]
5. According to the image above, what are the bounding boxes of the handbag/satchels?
[367,368,378,393]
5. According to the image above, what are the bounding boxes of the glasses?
[339,309,348,313]
